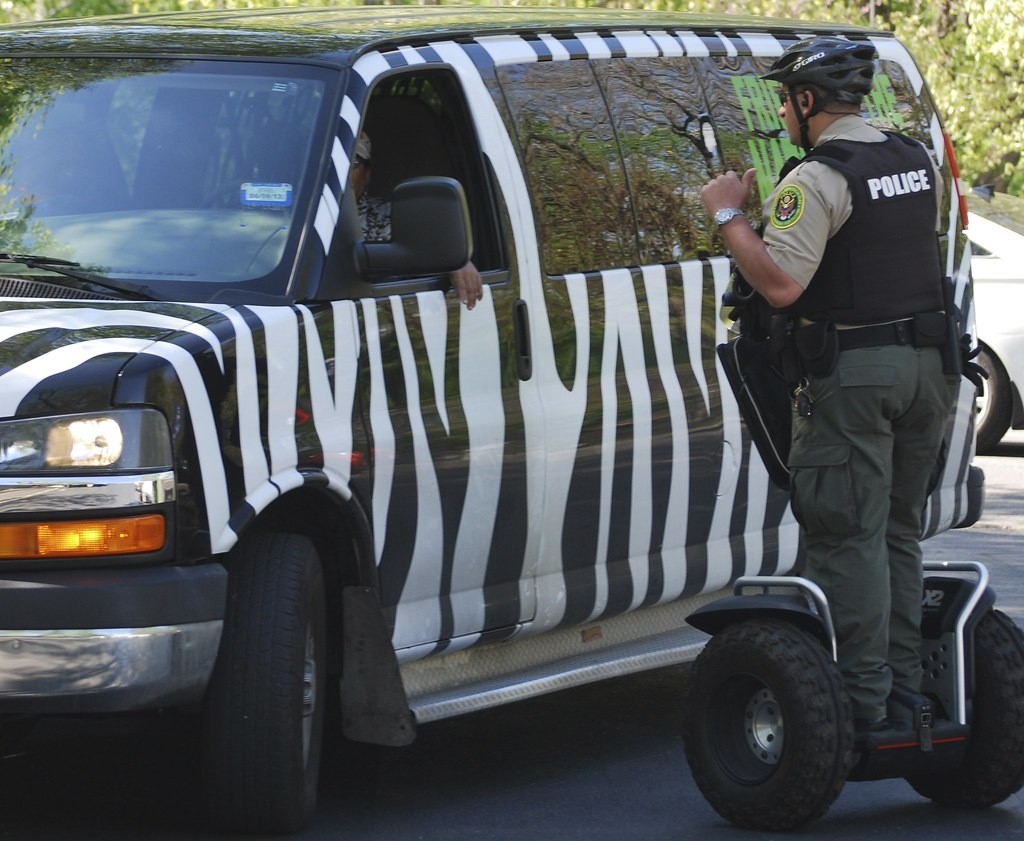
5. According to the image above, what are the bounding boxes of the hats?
[355,131,372,162]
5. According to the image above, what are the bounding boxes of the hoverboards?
[680,556,1024,837]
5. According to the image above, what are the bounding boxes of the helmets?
[756,36,876,105]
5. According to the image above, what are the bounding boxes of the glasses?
[775,86,805,105]
[353,157,366,169]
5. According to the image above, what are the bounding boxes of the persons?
[352,131,484,310]
[702,35,960,734]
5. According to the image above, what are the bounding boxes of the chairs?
[363,94,459,183]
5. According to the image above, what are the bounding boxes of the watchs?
[714,207,744,230]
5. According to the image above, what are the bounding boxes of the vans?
[0,19,982,839]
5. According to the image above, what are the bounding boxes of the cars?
[966,210,1024,456]
